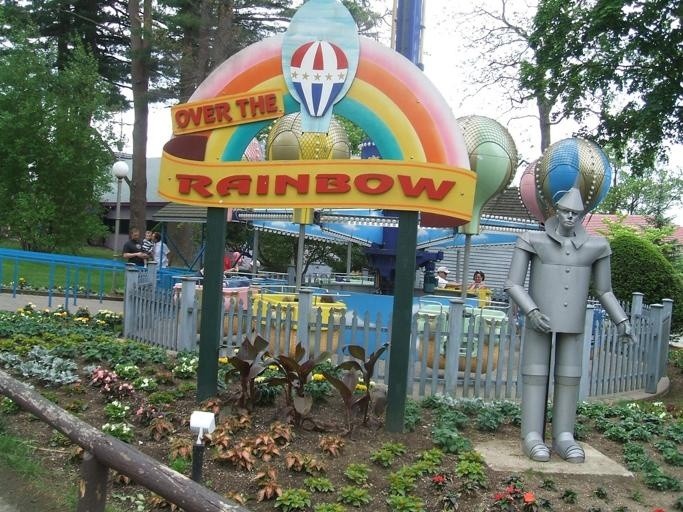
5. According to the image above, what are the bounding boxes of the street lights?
[108,161,129,297]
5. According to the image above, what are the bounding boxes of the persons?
[194,250,260,285]
[469,271,485,289]
[121,227,143,267]
[141,230,154,268]
[151,232,171,268]
[435,267,462,288]
[501,187,637,463]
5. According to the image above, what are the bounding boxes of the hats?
[233,250,243,263]
[437,266,451,275]
